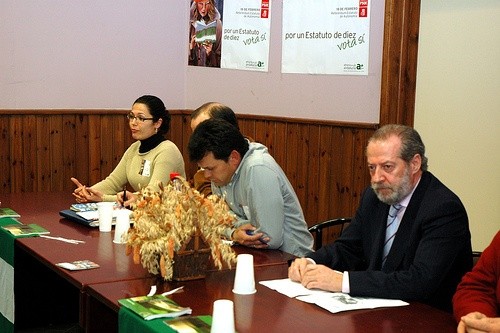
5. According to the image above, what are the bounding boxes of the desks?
[0,192,287,333]
[86,259,455,333]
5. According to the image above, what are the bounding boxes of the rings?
[253,244,255,248]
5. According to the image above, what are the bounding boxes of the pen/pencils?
[122,185,127,209]
[247,230,268,246]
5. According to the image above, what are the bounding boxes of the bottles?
[167,173,182,192]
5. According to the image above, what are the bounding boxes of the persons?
[70,94,186,208]
[188,0,222,68]
[188,118,316,257]
[451,231,500,333]
[189,101,256,200]
[288,123,473,311]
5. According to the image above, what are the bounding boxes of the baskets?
[158,175,213,281]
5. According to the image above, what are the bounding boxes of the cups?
[113,214,130,244]
[211,299,235,333]
[96,202,114,232]
[232,254,257,295]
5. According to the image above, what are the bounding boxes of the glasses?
[126,112,155,123]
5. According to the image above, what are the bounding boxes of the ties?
[381,204,402,270]
